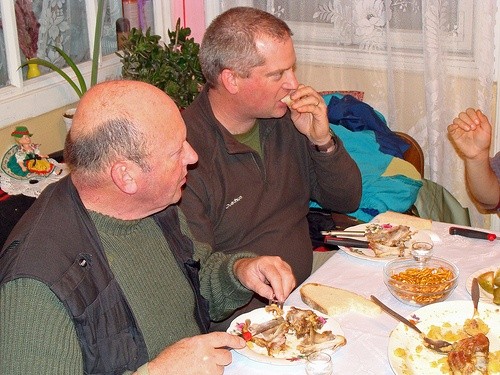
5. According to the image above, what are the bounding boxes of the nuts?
[388,267,454,304]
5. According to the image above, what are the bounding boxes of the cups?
[411,243,434,259]
[306,352,333,375]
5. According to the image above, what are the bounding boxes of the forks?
[267,286,285,316]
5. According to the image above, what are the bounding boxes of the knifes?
[215,318,282,350]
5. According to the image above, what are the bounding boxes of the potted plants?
[16,0,104,133]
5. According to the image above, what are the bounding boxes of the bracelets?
[137,362,150,375]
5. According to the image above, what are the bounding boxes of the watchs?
[308,128,339,152]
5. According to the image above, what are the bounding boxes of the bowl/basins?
[383,257,460,306]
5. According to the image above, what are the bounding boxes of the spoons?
[371,295,453,354]
[463,276,490,337]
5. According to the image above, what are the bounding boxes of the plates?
[388,301,500,375]
[466,267,500,303]
[337,223,434,261]
[226,306,343,366]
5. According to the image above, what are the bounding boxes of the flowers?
[13,0,41,59]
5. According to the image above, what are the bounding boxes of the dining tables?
[219,210,500,375]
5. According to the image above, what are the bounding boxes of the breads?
[300,283,382,317]
[281,94,300,106]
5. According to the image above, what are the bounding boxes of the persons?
[0,80,296,375]
[447,108,500,215]
[7,126,64,184]
[177,7,362,334]
